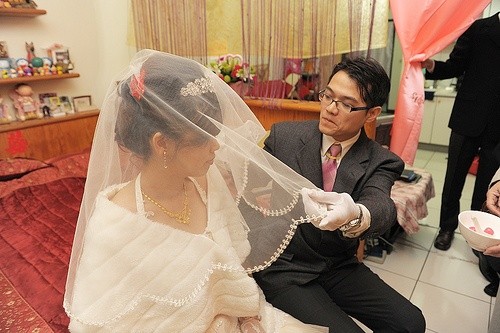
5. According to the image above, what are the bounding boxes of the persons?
[224,55,427,333]
[486,166,500,333]
[64,49,328,333]
[422,12,500,250]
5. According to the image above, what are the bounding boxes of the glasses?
[318,90,375,113]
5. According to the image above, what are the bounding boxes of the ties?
[322,144,342,192]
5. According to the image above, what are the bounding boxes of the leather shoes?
[434,228,454,250]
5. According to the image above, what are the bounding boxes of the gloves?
[300,187,360,231]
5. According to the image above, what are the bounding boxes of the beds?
[0,110,99,333]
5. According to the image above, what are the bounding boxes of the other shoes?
[484,281,499,297]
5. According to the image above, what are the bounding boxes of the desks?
[379,166,431,255]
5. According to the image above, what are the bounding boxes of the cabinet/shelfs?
[418,92,455,152]
[0,7,100,86]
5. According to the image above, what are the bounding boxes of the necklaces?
[142,182,192,225]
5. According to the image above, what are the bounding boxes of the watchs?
[340,207,363,232]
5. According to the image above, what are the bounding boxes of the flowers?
[209,56,258,84]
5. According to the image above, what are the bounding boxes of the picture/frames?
[39,93,57,105]
[73,95,91,112]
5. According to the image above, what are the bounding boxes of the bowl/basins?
[458,211,500,252]
